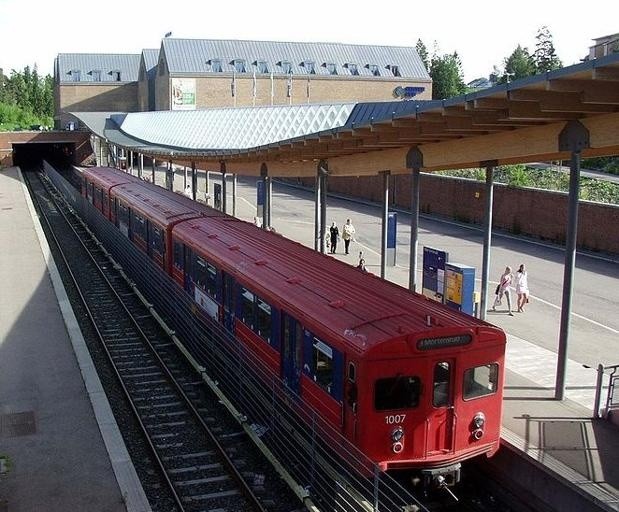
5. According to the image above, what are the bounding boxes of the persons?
[357,259,368,272]
[341,218,355,254]
[330,222,340,253]
[514,263,529,313]
[492,266,514,316]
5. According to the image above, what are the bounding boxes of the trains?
[77,165,512,495]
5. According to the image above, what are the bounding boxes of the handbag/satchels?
[495,296,501,306]
[495,285,500,294]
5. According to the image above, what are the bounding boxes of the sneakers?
[493,306,496,311]
[509,312,514,316]
[518,306,525,312]
